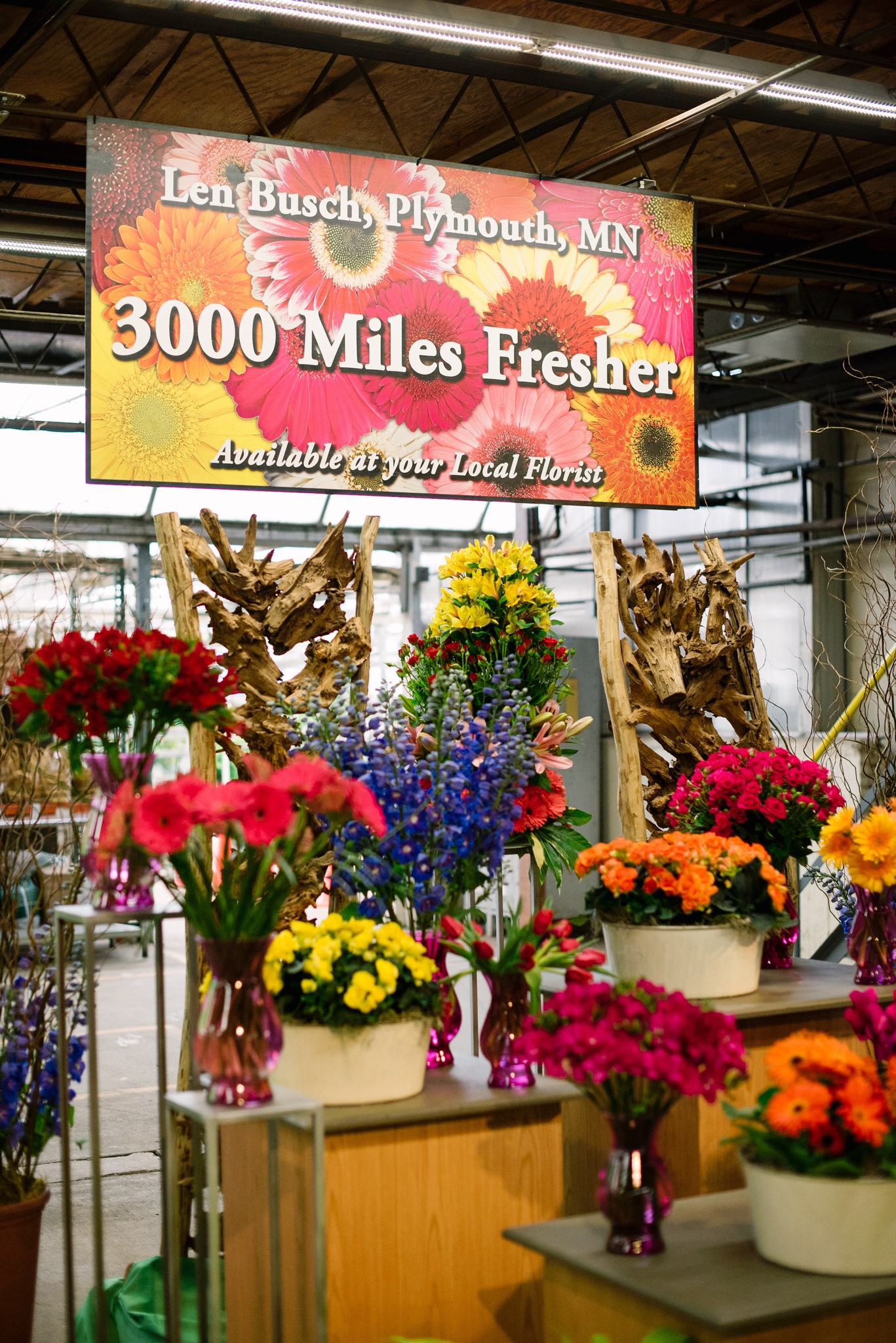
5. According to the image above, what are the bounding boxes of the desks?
[536,955,896,1214]
[502,1186,896,1343]
[215,1066,584,1343]
[0,802,90,858]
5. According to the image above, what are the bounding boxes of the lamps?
[163,0,896,121]
[0,220,86,262]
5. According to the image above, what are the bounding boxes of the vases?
[70,800,90,811]
[1,803,31,816]
[599,1113,669,1256]
[601,918,765,1000]
[0,1169,50,1343]
[481,971,537,1087]
[848,882,896,985]
[736,1152,896,1278]
[760,891,798,969]
[412,930,463,1070]
[266,1019,431,1105]
[192,931,283,1108]
[41,802,55,814]
[81,752,160,911]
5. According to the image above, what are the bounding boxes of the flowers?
[0,536,896,1203]
